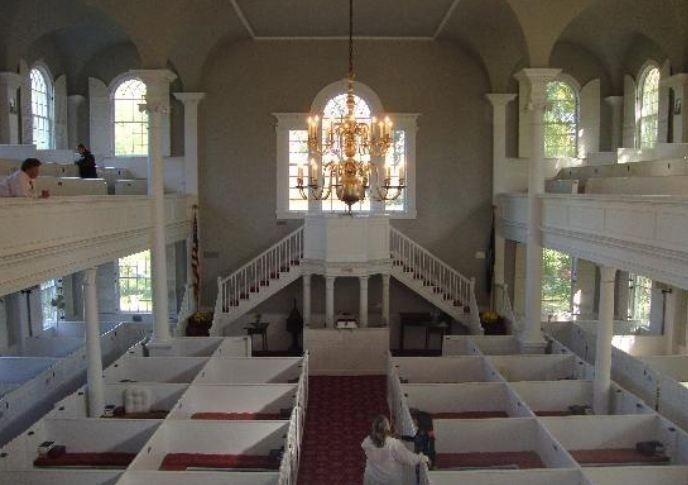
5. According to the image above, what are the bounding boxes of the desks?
[399,312,449,352]
[32,405,288,473]
[412,410,669,469]
[244,322,269,355]
[303,324,390,376]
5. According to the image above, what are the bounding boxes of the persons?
[75,142,98,180]
[394,410,437,469]
[360,414,432,485]
[0,156,50,199]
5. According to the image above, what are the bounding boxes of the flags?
[191,211,199,298]
[485,220,494,296]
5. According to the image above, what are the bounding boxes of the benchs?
[0,158,147,196]
[548,143,688,194]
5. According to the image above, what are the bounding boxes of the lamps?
[296,0,406,216]
